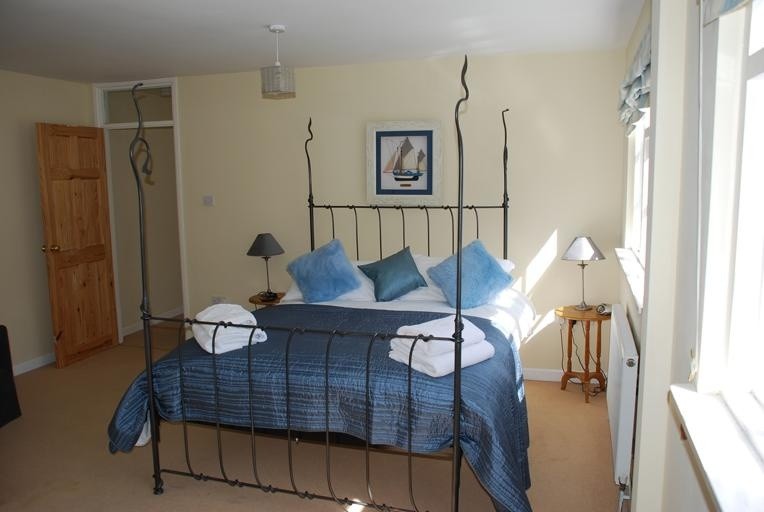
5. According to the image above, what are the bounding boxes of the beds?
[137,204,522,512]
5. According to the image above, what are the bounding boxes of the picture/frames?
[364,119,441,206]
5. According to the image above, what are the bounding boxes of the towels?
[191,303,267,355]
[388,314,495,378]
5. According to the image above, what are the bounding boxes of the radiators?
[606,303,639,512]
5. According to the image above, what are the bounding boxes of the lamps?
[259,24,295,96]
[560,237,605,311]
[246,233,284,302]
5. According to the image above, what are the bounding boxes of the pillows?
[279,237,515,310]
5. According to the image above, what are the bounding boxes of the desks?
[248,292,285,310]
[554,304,612,403]
